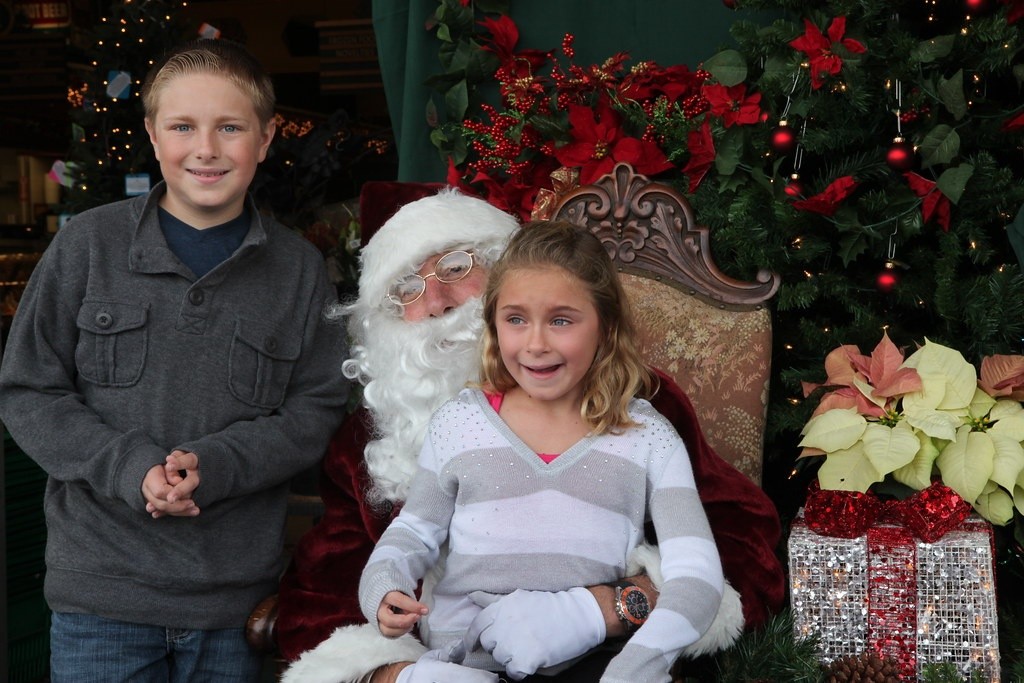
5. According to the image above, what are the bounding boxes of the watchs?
[602,579,650,640]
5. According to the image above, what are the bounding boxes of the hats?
[353,181,523,309]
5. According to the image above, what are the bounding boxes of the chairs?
[245,160,781,649]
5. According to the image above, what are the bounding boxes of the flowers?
[425,0,951,248]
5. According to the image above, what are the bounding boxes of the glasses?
[385,251,476,306]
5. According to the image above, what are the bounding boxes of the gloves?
[397,638,502,683]
[465,584,608,679]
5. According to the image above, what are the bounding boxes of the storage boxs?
[786,481,1001,683]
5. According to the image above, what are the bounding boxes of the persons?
[358,222,724,683]
[279,185,786,683]
[0,50,351,682]
